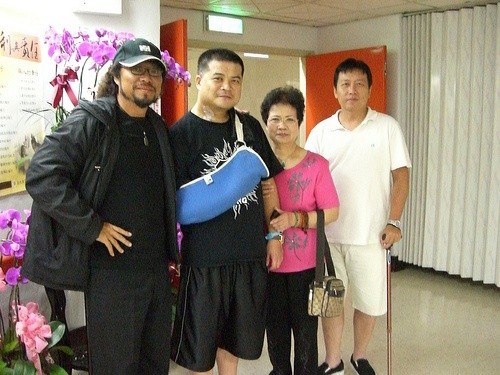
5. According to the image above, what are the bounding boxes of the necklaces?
[269,145,299,163]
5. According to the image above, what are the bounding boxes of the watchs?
[386,219,402,230]
[266,231,286,243]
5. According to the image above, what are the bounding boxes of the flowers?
[44,26,192,131]
[0,208,76,375]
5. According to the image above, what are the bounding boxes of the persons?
[19,37,176,375]
[299,58,411,375]
[260,84,339,375]
[166,48,286,375]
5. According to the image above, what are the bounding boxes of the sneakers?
[350,354,376,375]
[318,359,344,375]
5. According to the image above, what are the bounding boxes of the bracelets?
[293,210,310,234]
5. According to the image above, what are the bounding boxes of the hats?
[114,38,167,71]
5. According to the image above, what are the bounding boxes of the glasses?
[267,117,299,125]
[120,66,164,77]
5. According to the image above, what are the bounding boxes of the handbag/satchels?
[308,275,345,318]
